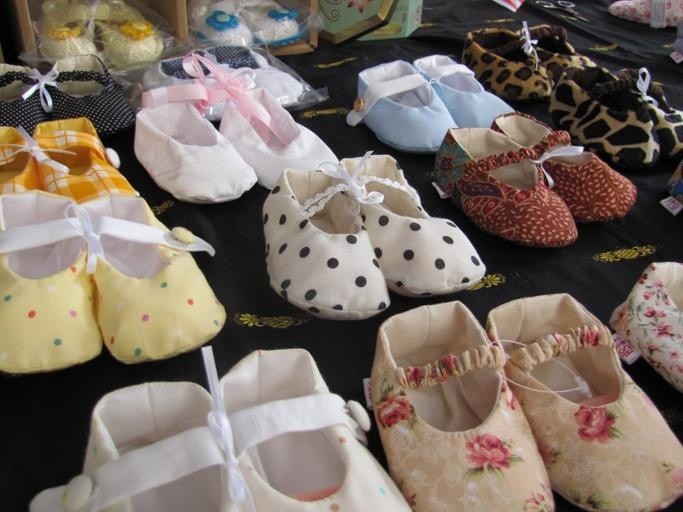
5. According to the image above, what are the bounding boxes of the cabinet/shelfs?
[17,0,318,70]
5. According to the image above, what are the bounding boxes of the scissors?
[536,0,590,23]
